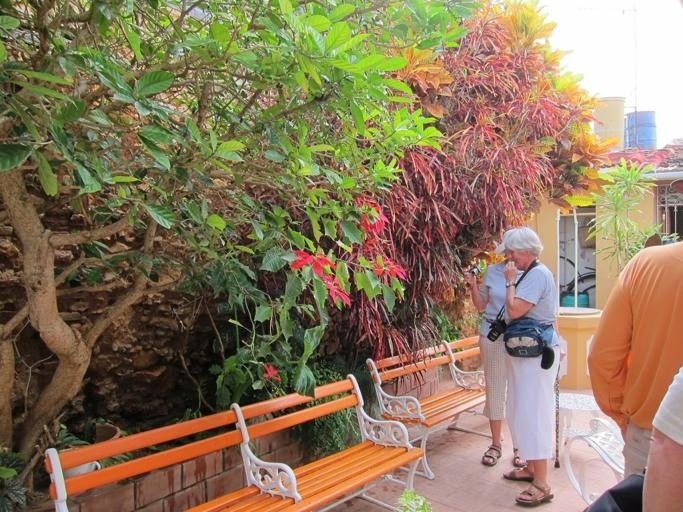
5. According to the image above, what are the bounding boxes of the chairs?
[559,416,626,512]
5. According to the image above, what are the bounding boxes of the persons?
[587,177,683,480]
[642,366,683,512]
[503,226,560,507]
[463,228,527,468]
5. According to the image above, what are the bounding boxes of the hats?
[494,229,517,256]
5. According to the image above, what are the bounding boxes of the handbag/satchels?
[503,318,554,357]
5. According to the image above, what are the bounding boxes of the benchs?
[45,375,427,512]
[366,334,505,480]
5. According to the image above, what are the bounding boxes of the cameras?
[484,316,507,342]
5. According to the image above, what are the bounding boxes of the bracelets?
[505,282,516,287]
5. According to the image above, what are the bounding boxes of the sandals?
[513,448,525,467]
[515,483,553,505]
[504,467,534,482]
[482,445,501,466]
